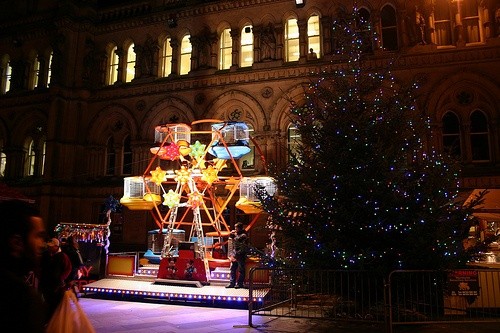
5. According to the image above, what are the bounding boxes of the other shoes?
[234,283,243,288]
[226,285,235,288]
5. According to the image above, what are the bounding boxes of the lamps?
[167,17,178,28]
[295,0,305,8]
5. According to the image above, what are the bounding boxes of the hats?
[47,238,60,247]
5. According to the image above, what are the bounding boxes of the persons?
[41,238,72,287]
[65,236,83,283]
[309,48,317,59]
[0,199,47,333]
[225,222,247,288]
[230,230,236,238]
